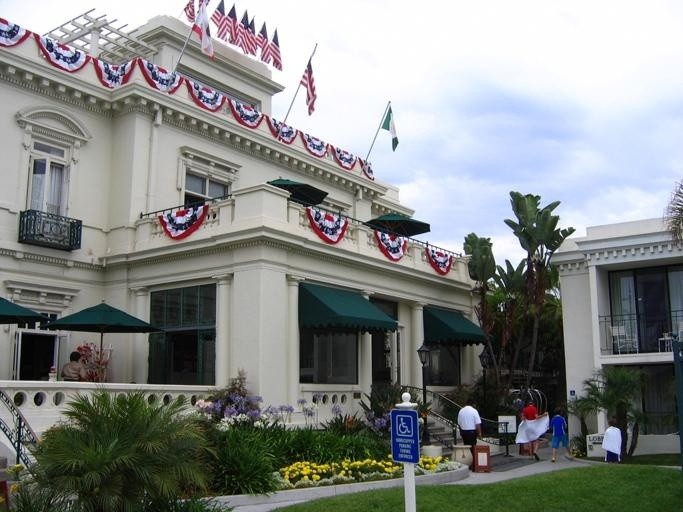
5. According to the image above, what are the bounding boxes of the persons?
[520,399,548,461]
[601,416,622,464]
[62,351,87,382]
[551,407,574,462]
[457,398,483,472]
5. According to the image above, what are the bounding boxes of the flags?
[185,0,284,72]
[300,58,317,117]
[380,104,399,152]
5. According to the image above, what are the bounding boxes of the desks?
[658,336,677,353]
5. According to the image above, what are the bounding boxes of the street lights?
[479,345,490,422]
[418,342,430,445]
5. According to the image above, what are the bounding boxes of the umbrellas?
[37,300,168,364]
[267,175,329,205]
[361,213,431,236]
[0,297,53,324]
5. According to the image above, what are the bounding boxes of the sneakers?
[531,451,540,462]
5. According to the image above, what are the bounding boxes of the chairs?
[607,326,639,355]
[676,321,682,352]
[60,375,82,381]
